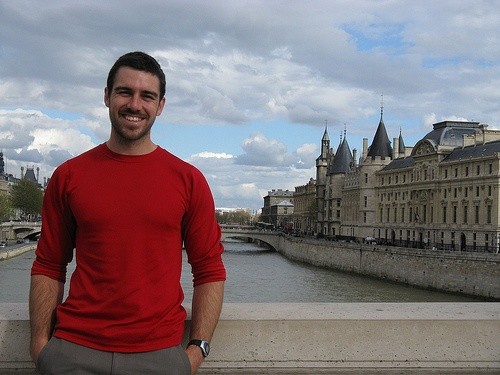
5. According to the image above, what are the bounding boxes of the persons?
[1,208,42,247]
[257,220,401,248]
[29,51,228,374]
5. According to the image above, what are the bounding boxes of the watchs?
[185,338,211,357]
